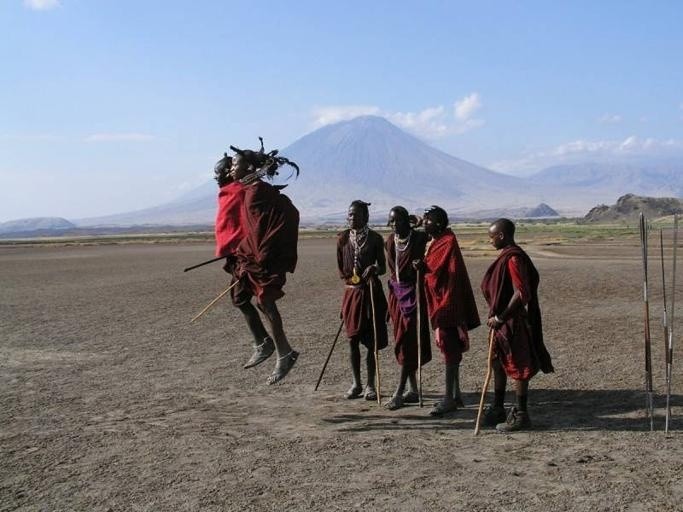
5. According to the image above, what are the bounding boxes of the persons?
[228,149,300,386]
[475,218,555,432]
[214,158,278,276]
[412,205,480,416]
[337,200,387,401]
[384,205,431,410]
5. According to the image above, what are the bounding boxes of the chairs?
[474,405,506,425]
[266,349,299,385]
[344,385,377,400]
[244,336,276,367]
[383,390,418,410]
[429,397,464,414]
[496,407,531,431]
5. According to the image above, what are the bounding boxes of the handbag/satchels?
[494,314,505,324]
